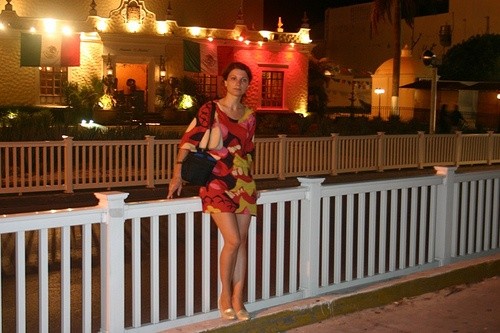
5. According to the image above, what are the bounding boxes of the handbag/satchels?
[180,150,217,187]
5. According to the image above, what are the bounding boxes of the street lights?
[374,88,384,118]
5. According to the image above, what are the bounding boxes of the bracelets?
[177,162,182,164]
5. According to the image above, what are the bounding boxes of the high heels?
[219,301,235,320]
[232,304,251,321]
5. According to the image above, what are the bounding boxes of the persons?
[167,62,256,321]
[450,105,468,128]
[439,104,451,129]
